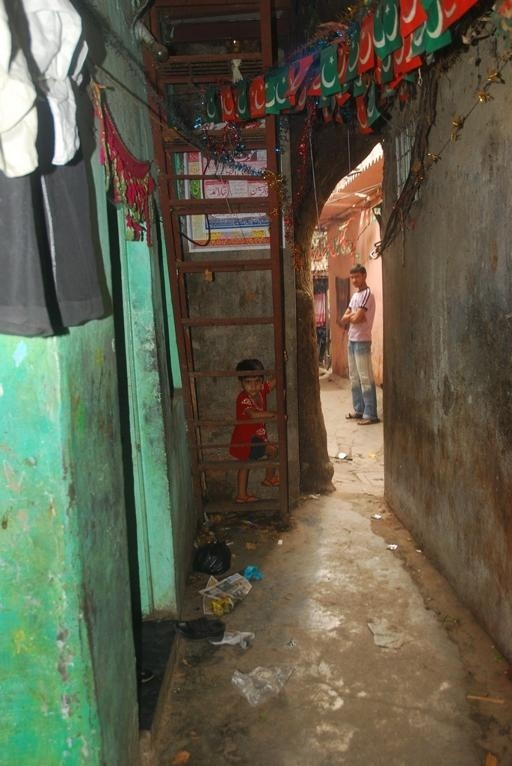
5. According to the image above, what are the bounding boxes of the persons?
[340,262,380,425]
[227,348,291,504]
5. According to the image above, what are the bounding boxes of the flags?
[311,232,361,265]
[203,0,477,134]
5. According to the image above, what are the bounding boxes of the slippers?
[344,411,380,426]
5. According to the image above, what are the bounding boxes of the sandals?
[235,475,282,504]
